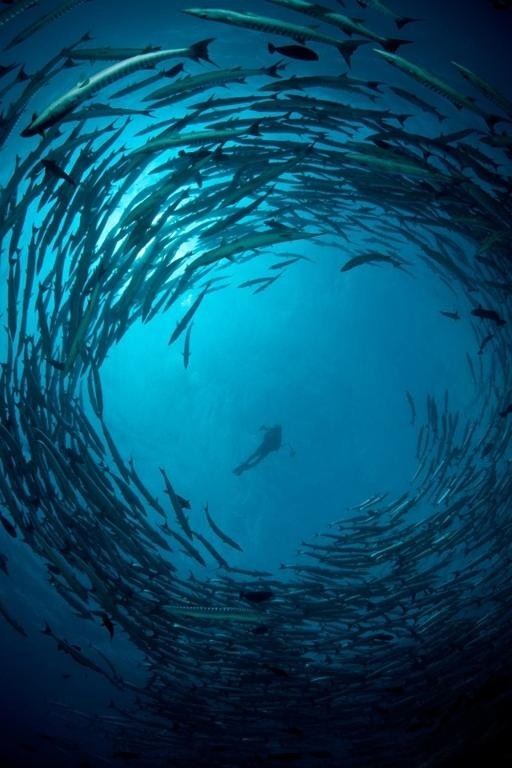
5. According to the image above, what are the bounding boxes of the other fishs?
[0,0,512,768]
